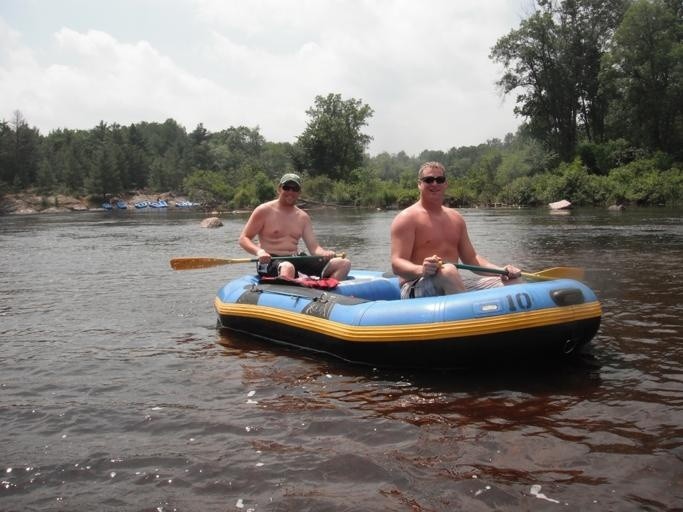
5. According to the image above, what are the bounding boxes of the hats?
[280,173,302,189]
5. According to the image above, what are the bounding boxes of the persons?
[390,161,524,302]
[238,172,352,282]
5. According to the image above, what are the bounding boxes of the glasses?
[281,185,300,192]
[419,175,446,184]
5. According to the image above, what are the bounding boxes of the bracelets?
[255,249,264,257]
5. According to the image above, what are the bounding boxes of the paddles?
[440,262,583,282]
[170,252,345,270]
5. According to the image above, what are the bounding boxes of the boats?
[211,267,604,372]
